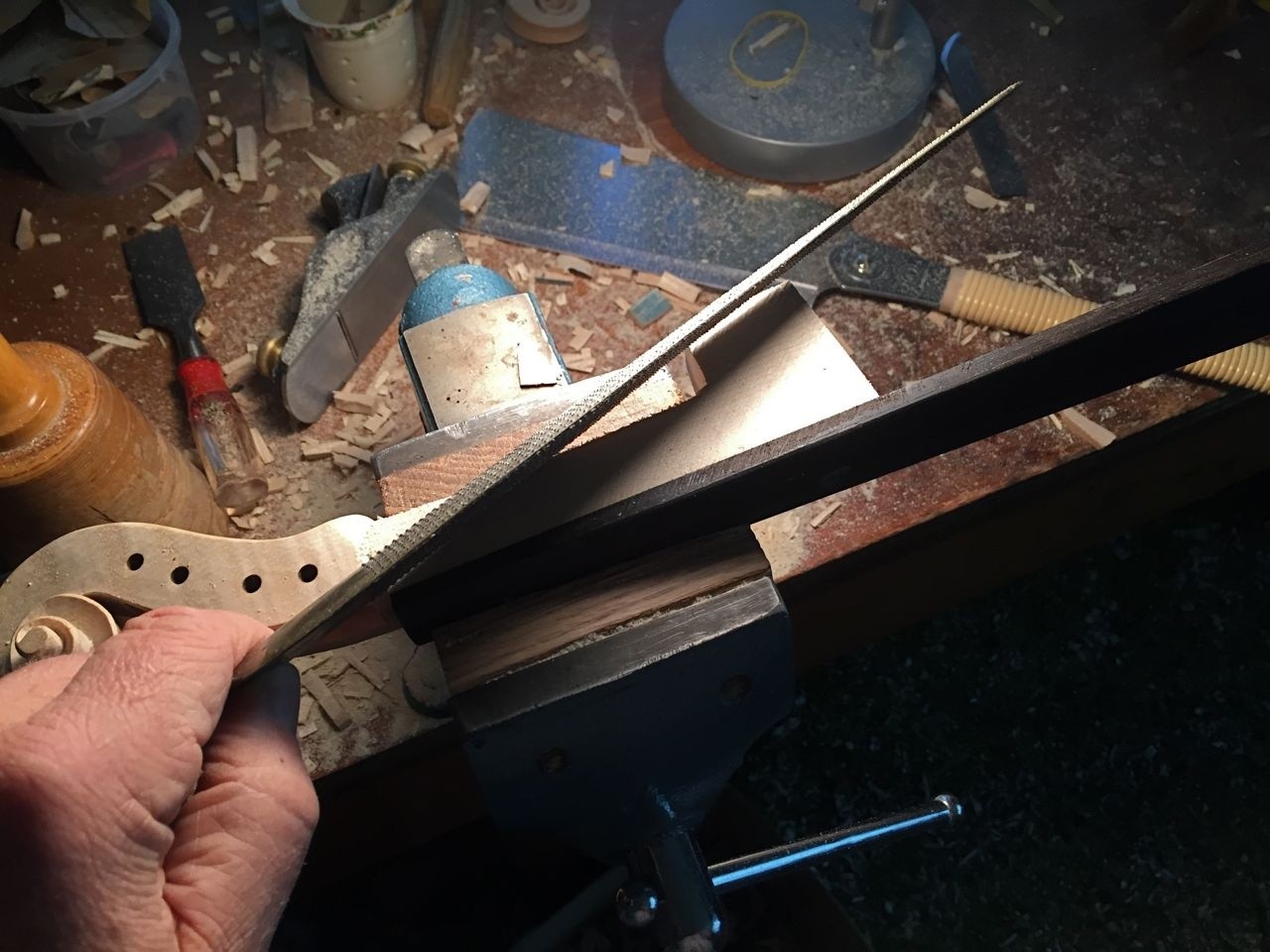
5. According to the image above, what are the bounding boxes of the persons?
[0,604,318,952]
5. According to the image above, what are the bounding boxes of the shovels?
[124,224,270,510]
